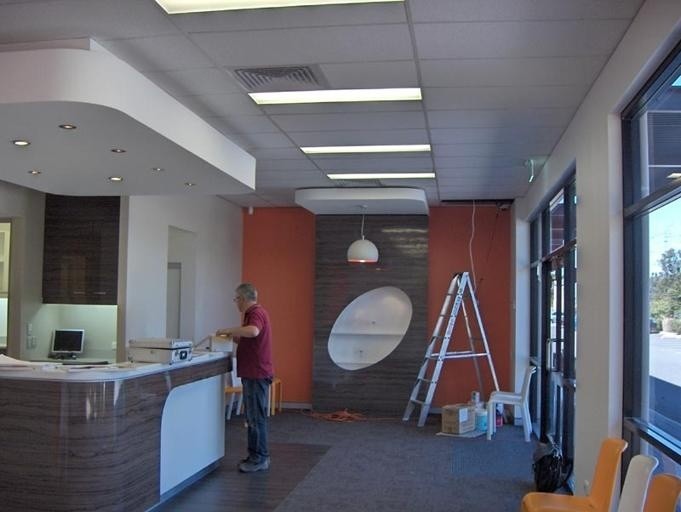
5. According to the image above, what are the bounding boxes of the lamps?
[345,203,381,264]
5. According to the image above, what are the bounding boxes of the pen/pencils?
[69,365,114,370]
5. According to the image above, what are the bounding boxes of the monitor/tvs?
[52,328,84,360]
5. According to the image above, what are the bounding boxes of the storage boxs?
[442,402,476,435]
[193,334,232,354]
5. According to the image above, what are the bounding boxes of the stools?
[223,375,282,421]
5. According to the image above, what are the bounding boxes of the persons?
[216,283,275,472]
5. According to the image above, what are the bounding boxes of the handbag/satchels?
[531,443,574,494]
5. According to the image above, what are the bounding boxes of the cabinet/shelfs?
[326,330,409,372]
[41,191,120,305]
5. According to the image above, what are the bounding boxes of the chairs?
[485,363,540,441]
[644,474,681,512]
[612,455,661,511]
[522,438,628,511]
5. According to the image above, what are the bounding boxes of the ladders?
[402,271,509,428]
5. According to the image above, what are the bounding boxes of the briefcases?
[126,336,194,364]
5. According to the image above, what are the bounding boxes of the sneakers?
[239,454,271,466]
[238,453,269,473]
